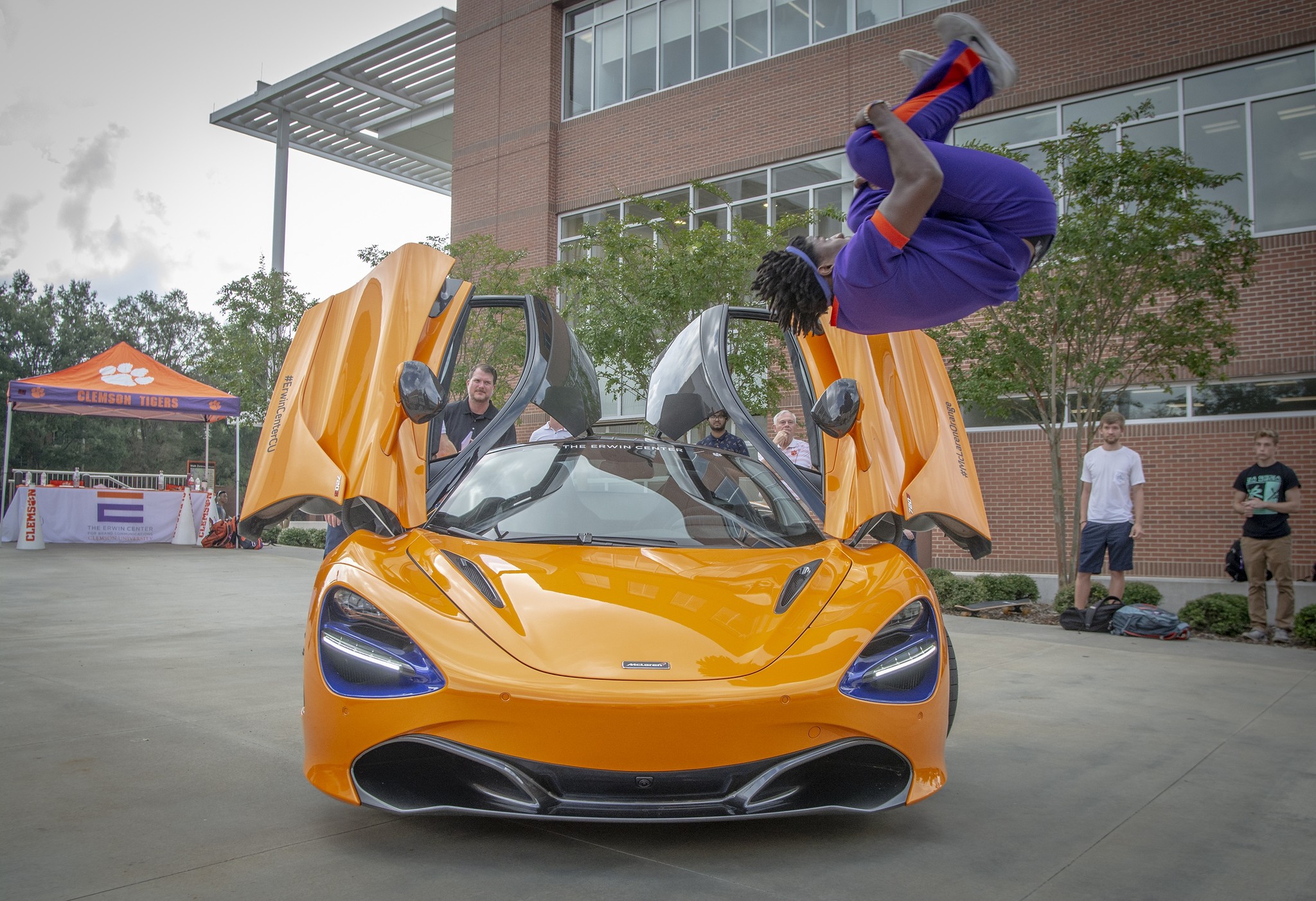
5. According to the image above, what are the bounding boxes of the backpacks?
[200,516,231,547]
[1222,537,1272,582]
[1111,602,1192,640]
[235,513,263,550]
[221,515,242,548]
[1059,596,1124,633]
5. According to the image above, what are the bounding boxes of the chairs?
[527,461,720,537]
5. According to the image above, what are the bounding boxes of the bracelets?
[1079,521,1086,525]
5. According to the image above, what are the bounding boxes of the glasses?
[709,415,728,421]
[775,420,795,426]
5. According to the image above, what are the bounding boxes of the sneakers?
[1241,630,1267,642]
[1272,629,1290,643]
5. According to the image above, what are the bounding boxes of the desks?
[0,485,218,543]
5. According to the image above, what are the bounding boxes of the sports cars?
[232,244,996,829]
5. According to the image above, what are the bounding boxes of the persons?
[748,13,1057,345]
[1231,428,1302,643]
[443,363,517,454]
[695,409,751,458]
[373,419,458,537]
[757,410,813,528]
[1074,411,1146,610]
[215,490,228,520]
[303,514,348,654]
[529,416,573,441]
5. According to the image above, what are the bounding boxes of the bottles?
[195,477,200,491]
[201,480,207,492]
[73,467,80,488]
[188,476,194,491]
[25,471,32,487]
[41,471,47,486]
[158,470,164,491]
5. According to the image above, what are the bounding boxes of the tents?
[0,341,240,549]
